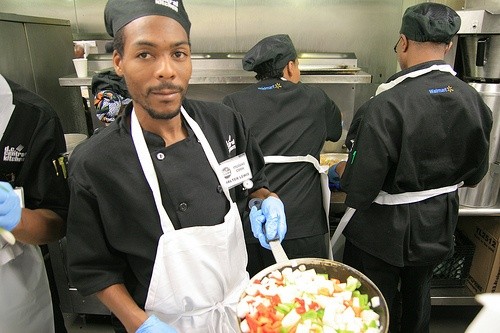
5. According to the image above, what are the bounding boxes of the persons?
[92,66,133,127]
[224,34,342,279]
[325,3,494,333]
[66,0,287,333]
[0,72,70,333]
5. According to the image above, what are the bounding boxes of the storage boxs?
[463,216,500,295]
[428,237,474,288]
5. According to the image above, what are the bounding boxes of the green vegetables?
[274,274,378,333]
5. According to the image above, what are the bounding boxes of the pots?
[321,129,350,153]
[236,197,389,333]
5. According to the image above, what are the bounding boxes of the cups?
[72,58,88,78]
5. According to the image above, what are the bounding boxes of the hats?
[104,0,191,41]
[400,3,461,45]
[243,34,297,80]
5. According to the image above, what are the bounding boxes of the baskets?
[429,229,476,287]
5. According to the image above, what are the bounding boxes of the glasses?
[393,37,404,53]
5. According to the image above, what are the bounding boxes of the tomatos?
[246,277,318,333]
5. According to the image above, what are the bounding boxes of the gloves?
[135,312,177,333]
[250,196,287,249]
[0,182,21,231]
[328,161,346,183]
